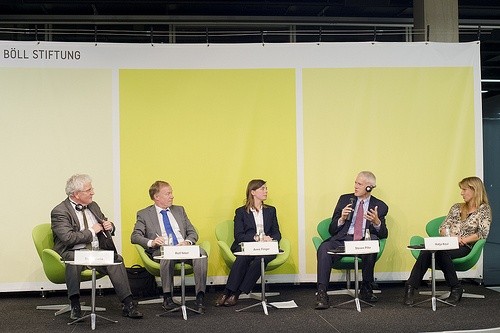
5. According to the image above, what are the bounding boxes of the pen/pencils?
[155,233,159,238]
[101,231,107,238]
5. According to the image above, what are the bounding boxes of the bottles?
[92,237,99,250]
[365,229,371,240]
[445,225,451,237]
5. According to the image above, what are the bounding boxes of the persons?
[50,174,143,322]
[315,171,388,309]
[402,177,493,305]
[131,181,208,313]
[214,180,282,306]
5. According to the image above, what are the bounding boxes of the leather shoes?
[194,300,207,313]
[122,304,143,319]
[71,306,82,320]
[215,294,226,306]
[445,287,463,304]
[404,285,414,305]
[163,301,179,311]
[224,294,238,305]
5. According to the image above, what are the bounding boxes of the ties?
[354,200,364,241]
[81,209,92,250]
[160,210,178,245]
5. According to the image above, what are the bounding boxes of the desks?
[327,250,380,312]
[406,246,460,311]
[232,251,286,316]
[153,255,208,320]
[60,260,123,330]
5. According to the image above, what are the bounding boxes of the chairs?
[409,216,487,299]
[136,241,211,306]
[214,220,290,301]
[31,223,106,316]
[312,218,386,299]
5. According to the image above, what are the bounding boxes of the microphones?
[347,198,354,221]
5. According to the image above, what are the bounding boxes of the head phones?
[365,186,376,192]
[69,200,86,211]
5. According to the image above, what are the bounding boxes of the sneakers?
[359,289,381,301]
[314,290,329,309]
